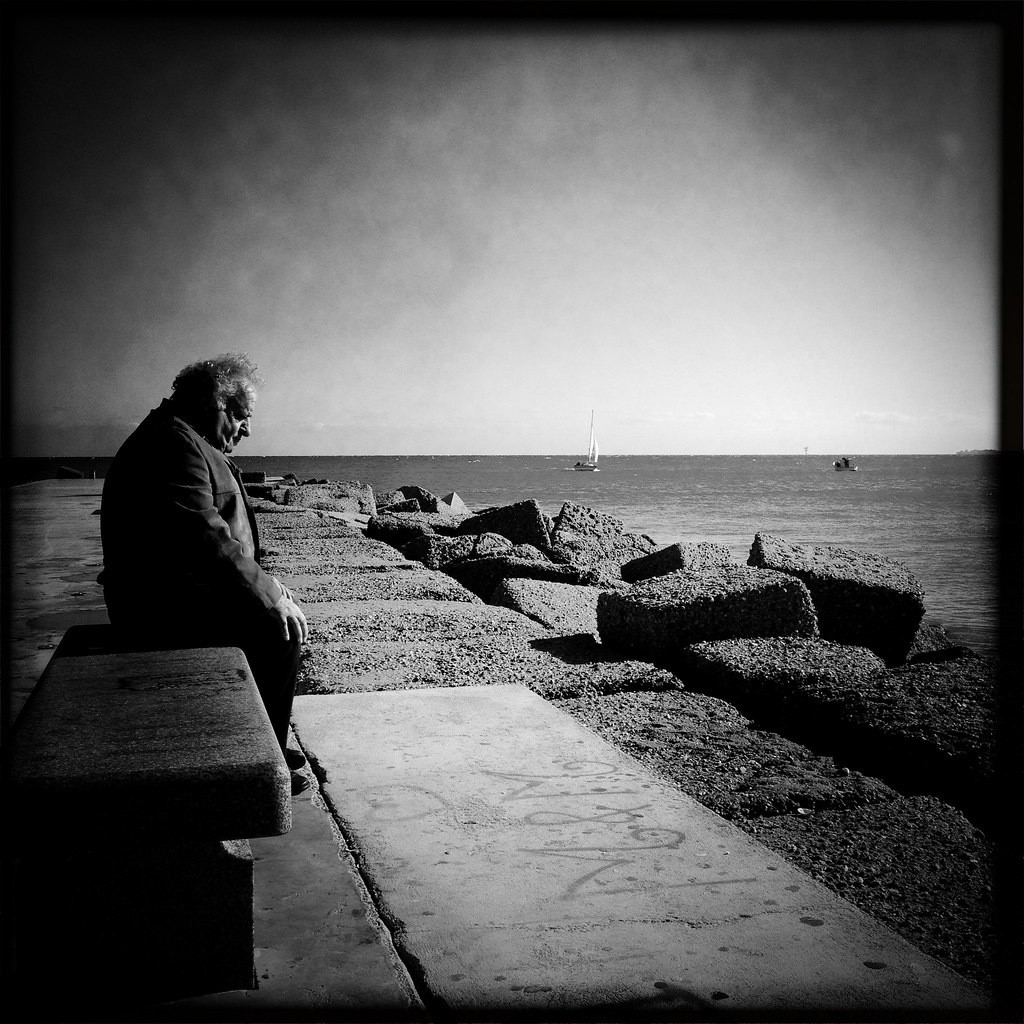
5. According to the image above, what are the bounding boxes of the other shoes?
[290,768,310,796]
[286,748,307,770]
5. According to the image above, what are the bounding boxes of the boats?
[832,457,859,471]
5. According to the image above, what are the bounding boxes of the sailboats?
[574,410,599,471]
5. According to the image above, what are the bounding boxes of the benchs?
[0,625,293,989]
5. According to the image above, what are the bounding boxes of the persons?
[96,350,310,795]
